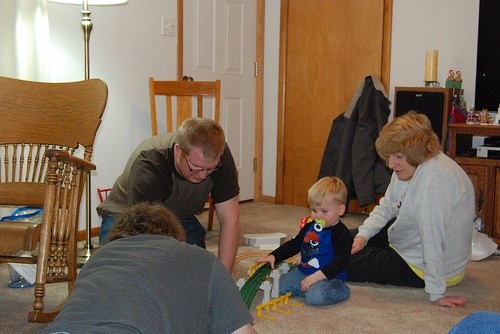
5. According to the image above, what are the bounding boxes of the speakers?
[394,87,464,154]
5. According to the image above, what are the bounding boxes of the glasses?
[184,154,222,173]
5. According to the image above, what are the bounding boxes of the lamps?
[49,0,129,269]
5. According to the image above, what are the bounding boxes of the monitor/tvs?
[474,0,500,113]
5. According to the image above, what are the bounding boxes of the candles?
[424,49,439,82]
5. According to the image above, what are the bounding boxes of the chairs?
[0,75,109,323]
[148,77,222,231]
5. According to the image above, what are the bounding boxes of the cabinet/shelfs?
[394,87,500,249]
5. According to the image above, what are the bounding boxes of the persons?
[256,177,353,306]
[351,111,475,309]
[96,117,240,276]
[40,202,258,334]
